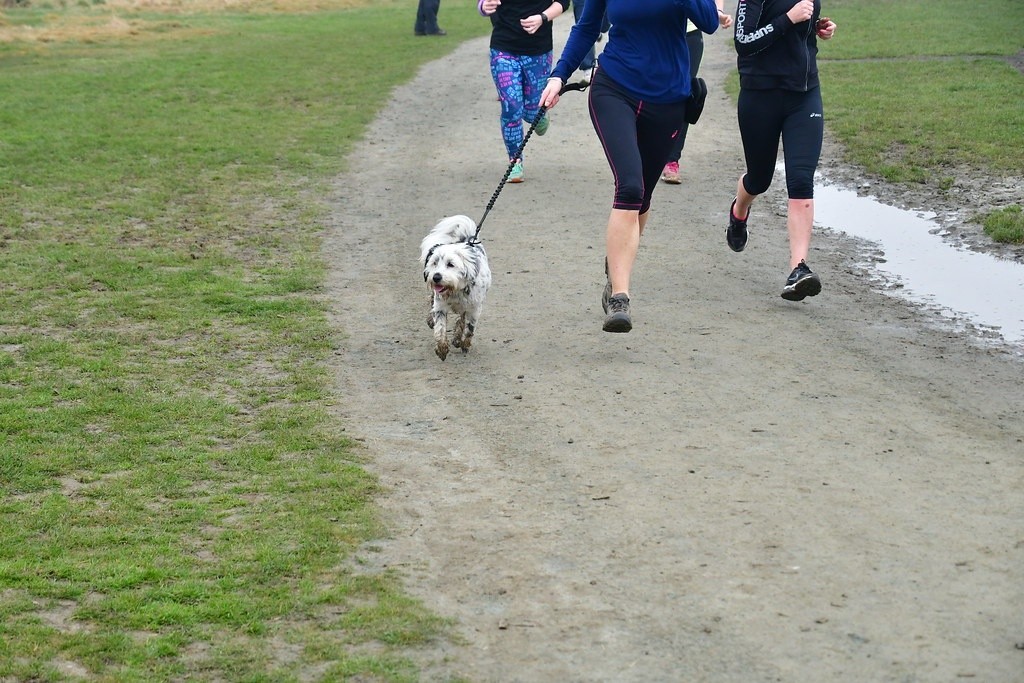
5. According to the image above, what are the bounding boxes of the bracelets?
[547,78,564,86]
[718,10,724,14]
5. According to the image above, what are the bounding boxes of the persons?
[536,0,719,334]
[477,0,571,183]
[572,0,611,70]
[725,0,837,330]
[660,0,733,186]
[413,0,447,36]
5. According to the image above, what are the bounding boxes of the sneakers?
[506,162,525,183]
[726,195,751,252]
[603,293,632,333]
[601,256,612,315]
[534,111,550,136]
[661,161,682,183]
[781,263,822,301]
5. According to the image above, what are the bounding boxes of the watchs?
[539,12,548,25]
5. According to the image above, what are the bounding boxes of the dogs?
[417,215,491,363]
